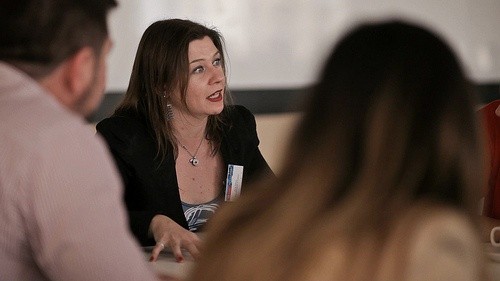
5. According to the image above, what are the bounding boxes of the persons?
[170,129,207,166]
[94,19,279,265]
[0,0,162,280]
[181,19,492,281]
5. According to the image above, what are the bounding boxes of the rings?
[156,241,164,247]
